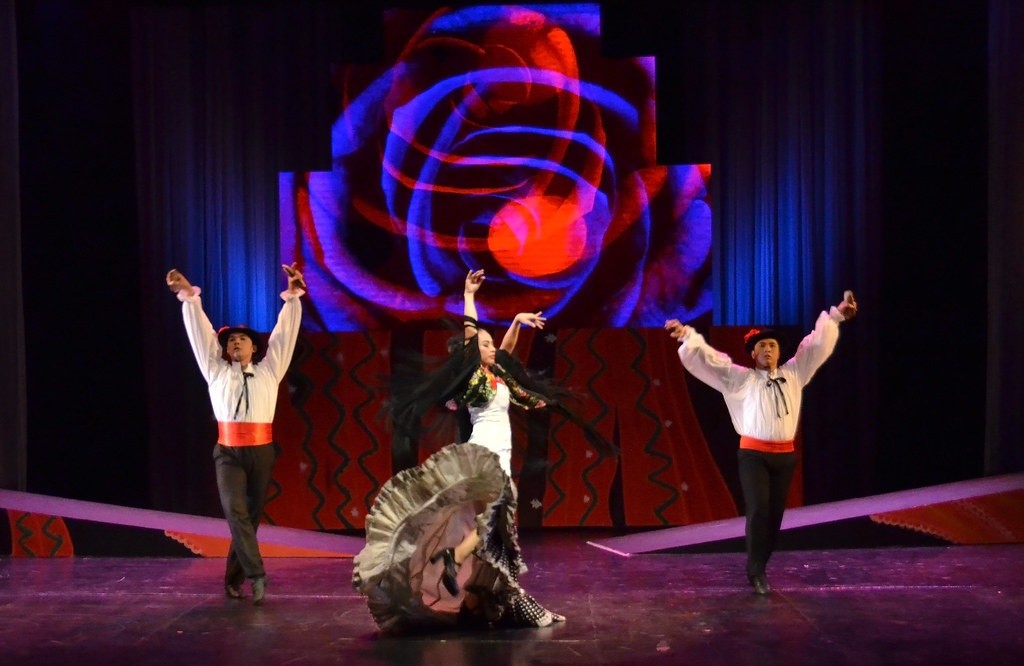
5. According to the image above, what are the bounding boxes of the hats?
[744,327,781,354]
[217,324,266,362]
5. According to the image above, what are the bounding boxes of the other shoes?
[752,573,772,596]
[251,576,268,605]
[225,584,240,598]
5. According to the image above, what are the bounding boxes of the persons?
[167,262,306,605]
[661,289,858,595]
[431,268,547,597]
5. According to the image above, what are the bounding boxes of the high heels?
[430,547,462,596]
